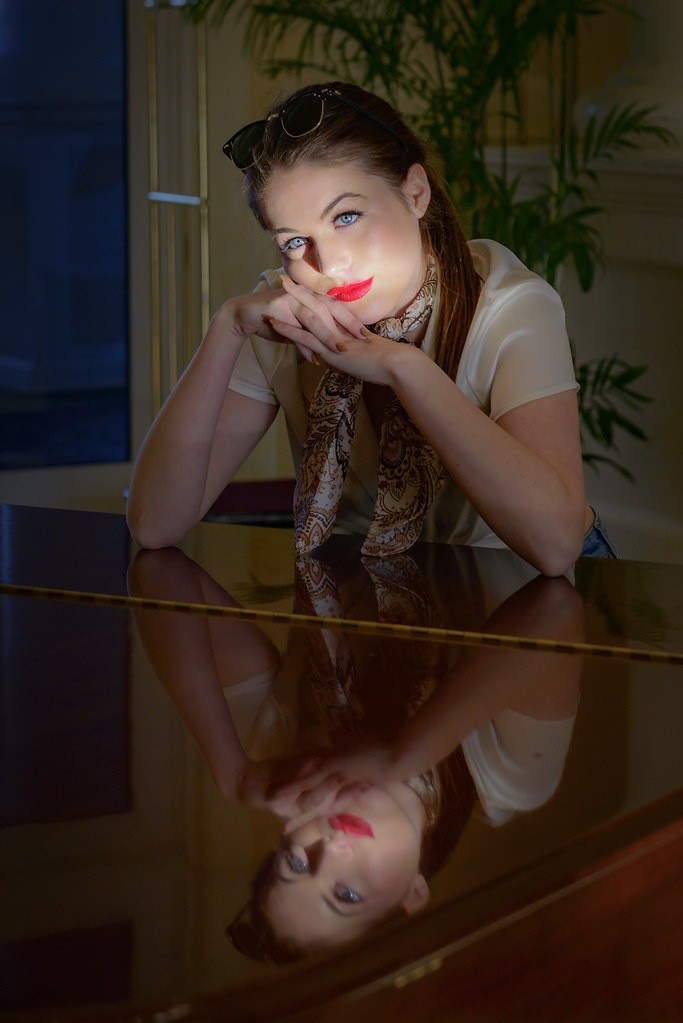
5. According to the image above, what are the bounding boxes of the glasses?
[222,903,310,974]
[222,85,413,174]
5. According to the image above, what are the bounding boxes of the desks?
[0,502,683,1023]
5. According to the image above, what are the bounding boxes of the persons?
[123,82,619,576]
[127,546,589,970]
